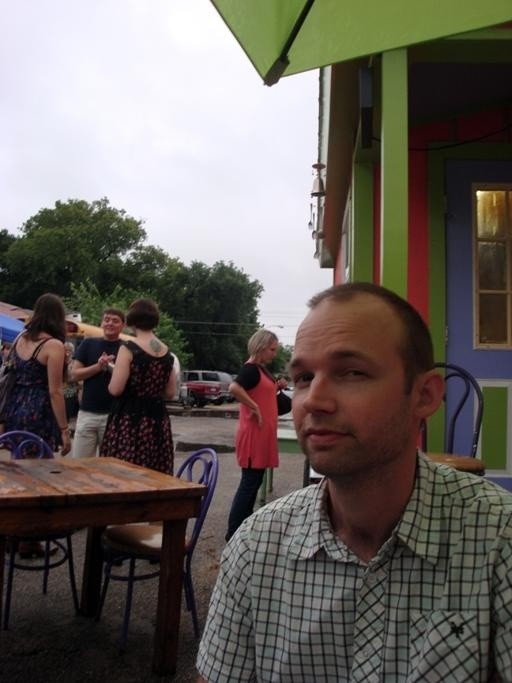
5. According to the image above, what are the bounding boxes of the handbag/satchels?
[1,327,29,424]
[275,380,293,415]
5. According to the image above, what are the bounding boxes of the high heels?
[18,541,59,560]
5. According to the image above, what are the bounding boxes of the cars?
[279,379,297,400]
[181,367,242,406]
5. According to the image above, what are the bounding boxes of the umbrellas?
[211,0,512,86]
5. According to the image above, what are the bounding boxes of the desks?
[0,456,207,683]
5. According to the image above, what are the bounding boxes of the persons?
[104,297,178,482]
[1,293,71,565]
[1,332,85,433]
[64,304,126,462]
[195,281,512,683]
[225,331,288,543]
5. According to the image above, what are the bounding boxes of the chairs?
[98,448,218,655]
[415,362,485,476]
[0,431,80,623]
